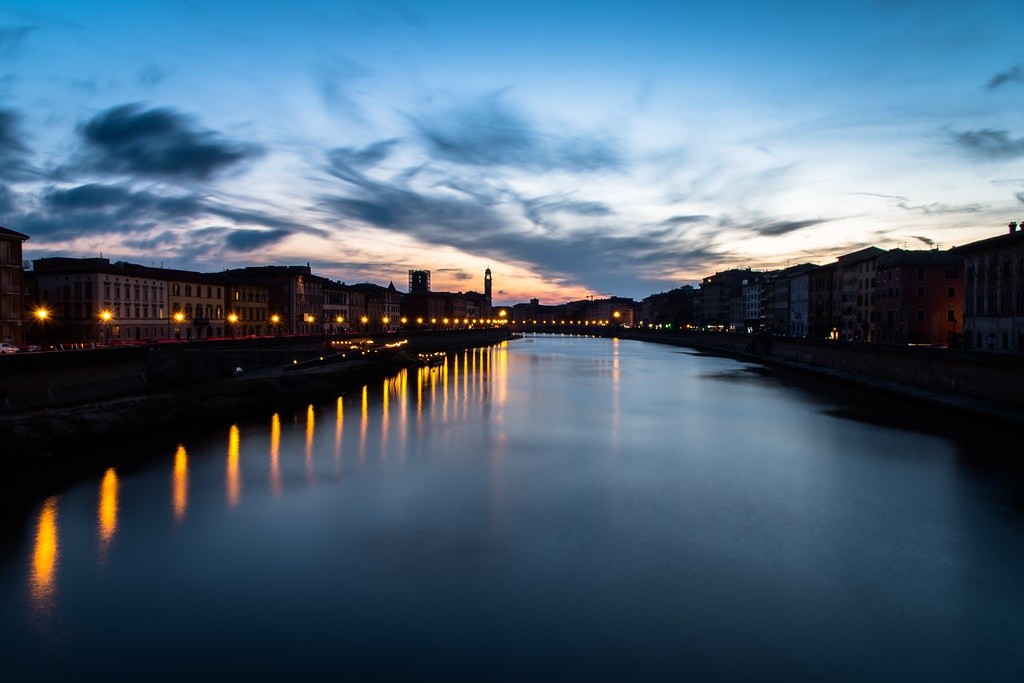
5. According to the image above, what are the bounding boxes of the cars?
[0,342,21,355]
